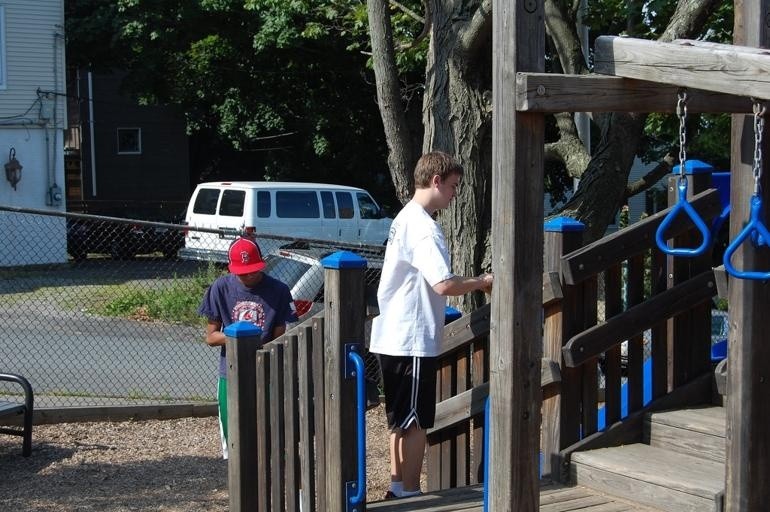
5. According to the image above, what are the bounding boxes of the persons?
[368,152,494,502]
[198,238,301,462]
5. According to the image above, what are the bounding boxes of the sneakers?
[384,490,398,499]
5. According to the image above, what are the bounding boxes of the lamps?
[5,147,23,191]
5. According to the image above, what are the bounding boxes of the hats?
[228,236,266,276]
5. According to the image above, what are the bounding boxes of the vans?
[178,182,395,266]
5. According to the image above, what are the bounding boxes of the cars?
[65,202,182,262]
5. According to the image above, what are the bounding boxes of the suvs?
[255,241,388,408]
